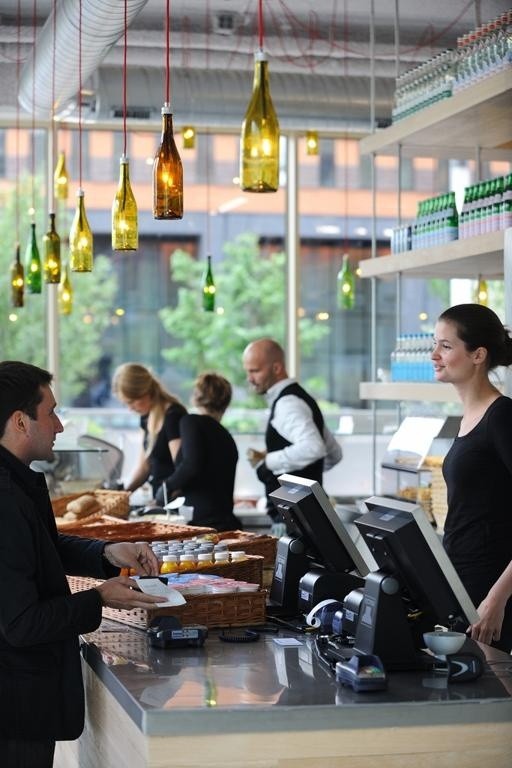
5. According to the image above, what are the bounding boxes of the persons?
[0,358,171,767]
[149,371,244,533]
[430,303,511,656]
[242,338,344,537]
[112,361,189,511]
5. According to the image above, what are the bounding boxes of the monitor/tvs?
[326,495,482,668]
[266,473,371,633]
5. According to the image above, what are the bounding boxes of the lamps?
[112,0,141,252]
[237,1,281,192]
[153,1,184,219]
[181,14,197,152]
[304,0,320,159]
[9,0,95,318]
[200,1,219,315]
[335,0,357,310]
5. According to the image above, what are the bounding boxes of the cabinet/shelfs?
[379,412,461,528]
[356,62,512,404]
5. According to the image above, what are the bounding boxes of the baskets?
[51,491,278,630]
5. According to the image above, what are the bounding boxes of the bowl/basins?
[424,630,464,658]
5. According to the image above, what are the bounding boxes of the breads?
[54,494,103,523]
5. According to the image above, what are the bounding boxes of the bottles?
[390,9,511,121]
[136,539,244,573]
[472,276,487,308]
[240,50,280,192]
[9,105,186,323]
[409,192,457,249]
[456,173,510,240]
[390,331,442,383]
[334,254,356,309]
[201,253,216,313]
